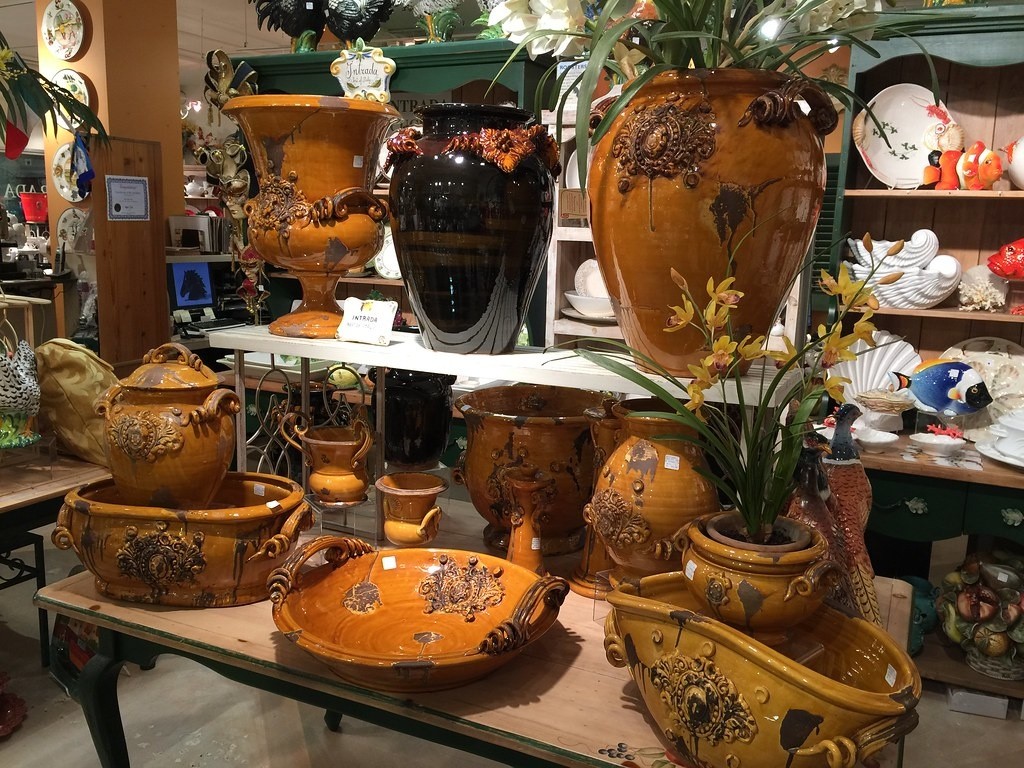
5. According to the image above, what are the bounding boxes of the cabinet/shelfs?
[31,4,1024,703]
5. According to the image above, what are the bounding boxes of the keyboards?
[188,318,246,331]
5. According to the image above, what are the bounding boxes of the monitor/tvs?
[167,263,216,315]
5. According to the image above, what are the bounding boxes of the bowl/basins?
[564,291,615,316]
[854,428,900,454]
[909,432,966,456]
[813,423,860,440]
[988,414,1024,461]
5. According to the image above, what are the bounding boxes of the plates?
[974,439,1024,468]
[852,83,964,189]
[560,308,616,322]
[376,234,402,280]
[824,324,1023,442]
[566,148,581,189]
[574,258,608,297]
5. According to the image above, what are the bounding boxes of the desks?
[32,537,695,768]
[0,450,115,668]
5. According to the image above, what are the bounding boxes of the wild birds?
[819,402,884,632]
[788,443,860,616]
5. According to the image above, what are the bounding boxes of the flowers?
[485,0,943,200]
[542,207,907,543]
[0,28,116,153]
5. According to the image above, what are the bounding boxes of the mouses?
[244,318,254,324]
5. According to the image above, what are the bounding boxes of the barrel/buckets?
[20,193,47,223]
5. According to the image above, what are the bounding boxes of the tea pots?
[184,179,204,197]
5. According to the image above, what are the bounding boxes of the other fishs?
[885,358,994,417]
[924,145,1001,188]
[985,237,1024,284]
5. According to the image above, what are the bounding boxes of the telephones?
[45,242,72,278]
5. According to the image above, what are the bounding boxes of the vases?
[706,513,811,552]
[382,103,562,353]
[587,67,839,379]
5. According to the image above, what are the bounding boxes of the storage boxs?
[946,685,1009,719]
